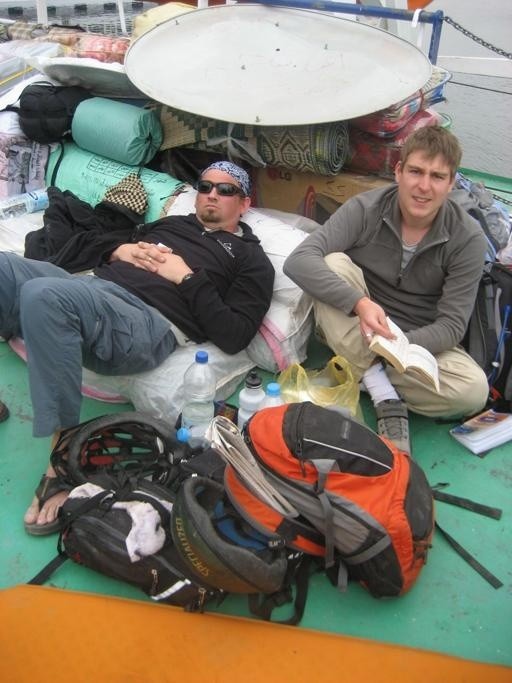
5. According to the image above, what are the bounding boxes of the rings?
[149,257,153,262]
[364,333,372,337]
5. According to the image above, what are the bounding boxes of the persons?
[0,159,276,536]
[281,121,491,454]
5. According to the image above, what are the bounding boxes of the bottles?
[0,187,49,218]
[176,351,285,449]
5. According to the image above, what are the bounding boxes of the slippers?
[24,472,78,535]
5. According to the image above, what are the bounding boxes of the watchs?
[181,273,196,282]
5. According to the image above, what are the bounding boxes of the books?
[449,408,512,455]
[368,315,440,394]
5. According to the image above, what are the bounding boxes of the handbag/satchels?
[57,475,229,611]
[462,259,512,414]
[18,85,90,145]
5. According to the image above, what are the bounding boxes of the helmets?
[172,476,290,594]
[49,410,191,491]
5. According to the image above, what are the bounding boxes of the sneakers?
[374,390,411,456]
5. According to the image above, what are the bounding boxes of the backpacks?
[224,402,434,600]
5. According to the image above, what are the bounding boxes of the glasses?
[196,180,242,197]
